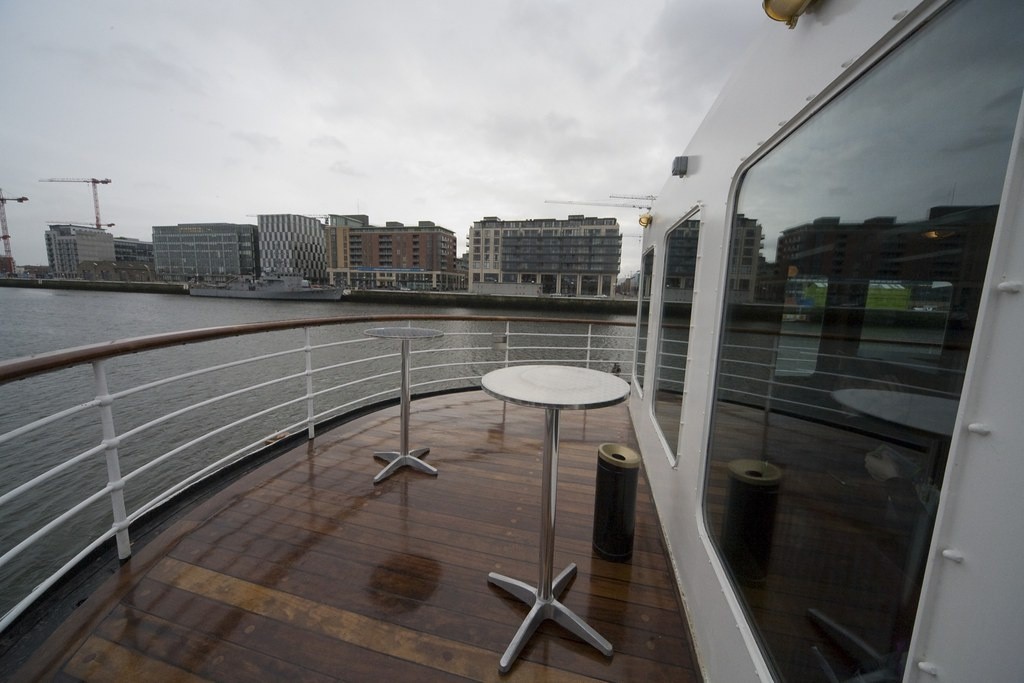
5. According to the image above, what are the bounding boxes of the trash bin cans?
[302,280,309,288]
[48,273,54,279]
[721,459,785,609]
[591,443,641,562]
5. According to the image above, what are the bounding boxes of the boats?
[188,271,342,301]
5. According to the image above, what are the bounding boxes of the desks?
[363,327,444,484]
[806,388,961,683]
[478,366,633,671]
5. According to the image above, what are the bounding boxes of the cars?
[913,305,935,312]
[593,294,608,298]
[550,292,561,296]
[568,294,577,297]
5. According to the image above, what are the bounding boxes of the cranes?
[0,187,29,256]
[543,195,658,212]
[38,176,116,229]
